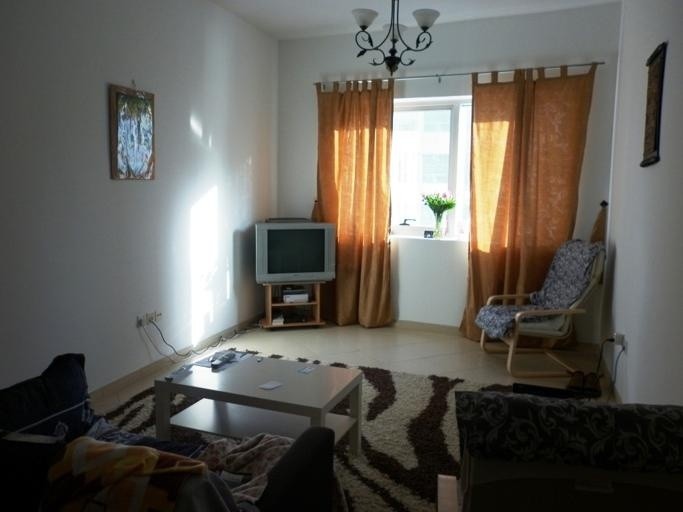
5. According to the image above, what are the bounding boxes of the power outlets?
[611,333,624,346]
[147,311,155,324]
[137,314,146,327]
[621,341,627,354]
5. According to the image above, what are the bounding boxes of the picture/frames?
[109,83,156,181]
[640,42,668,166]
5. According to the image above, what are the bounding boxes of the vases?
[433,214,444,238]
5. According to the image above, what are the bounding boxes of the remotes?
[211,350,234,371]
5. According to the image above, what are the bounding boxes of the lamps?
[352,0,440,77]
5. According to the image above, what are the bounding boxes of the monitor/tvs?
[254,218,338,285]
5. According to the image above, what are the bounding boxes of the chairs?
[480,239,605,378]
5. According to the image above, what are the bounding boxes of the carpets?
[97,348,510,512]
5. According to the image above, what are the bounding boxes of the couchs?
[0,353,341,512]
[451,383,681,512]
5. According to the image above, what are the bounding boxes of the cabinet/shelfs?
[261,280,327,328]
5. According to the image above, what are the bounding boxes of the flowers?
[420,193,455,231]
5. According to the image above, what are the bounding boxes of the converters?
[608,331,626,348]
